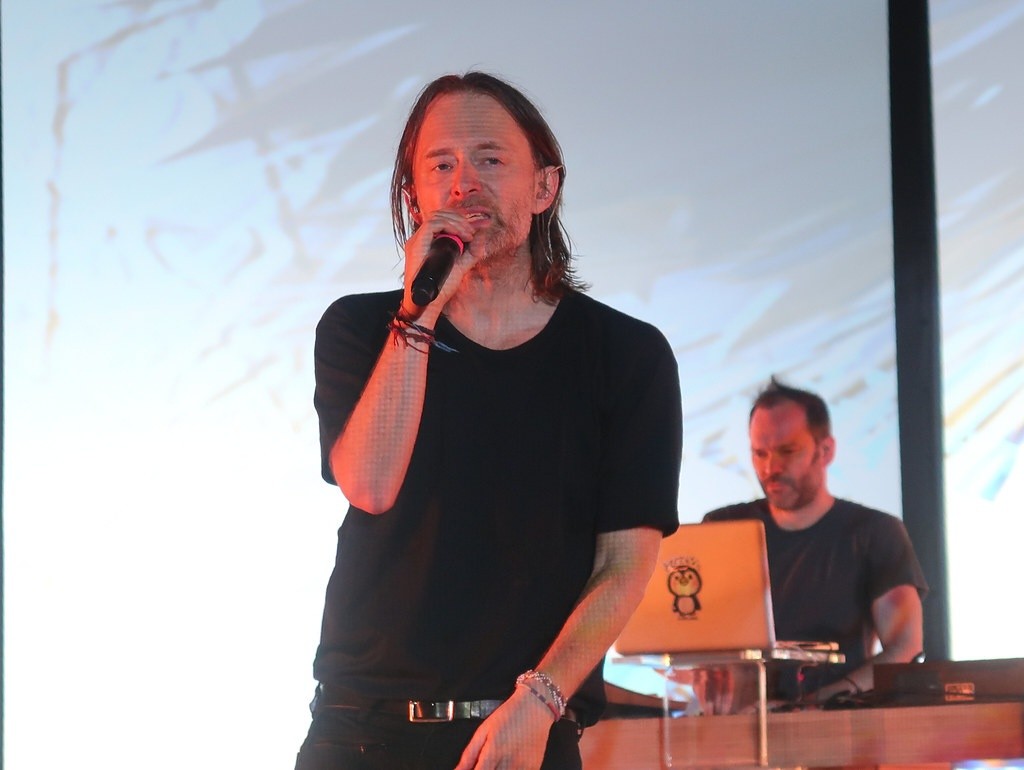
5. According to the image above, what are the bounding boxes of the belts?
[314,683,580,725]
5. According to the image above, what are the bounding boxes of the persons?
[293,72,683,770]
[655,377,930,712]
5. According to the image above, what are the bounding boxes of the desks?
[579,701,1024,770]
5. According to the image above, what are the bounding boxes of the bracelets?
[386,310,460,357]
[516,668,567,721]
[845,677,861,692]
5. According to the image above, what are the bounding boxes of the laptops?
[613,519,840,655]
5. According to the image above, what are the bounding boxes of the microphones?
[411,232,466,306]
